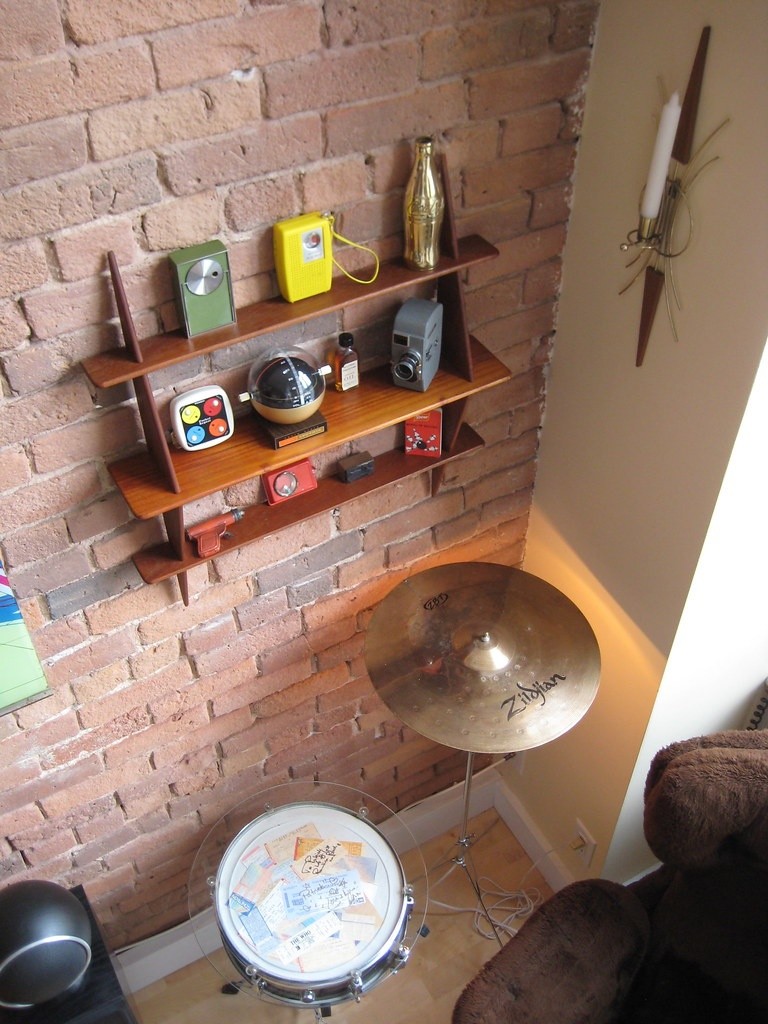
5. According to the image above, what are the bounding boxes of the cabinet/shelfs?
[73,152,514,608]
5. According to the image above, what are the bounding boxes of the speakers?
[0,880,92,1009]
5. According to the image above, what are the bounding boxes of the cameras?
[271,207,337,304]
[259,457,318,507]
[167,238,238,340]
[337,450,376,484]
[390,295,444,392]
[404,407,442,458]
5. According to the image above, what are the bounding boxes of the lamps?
[617,25,733,375]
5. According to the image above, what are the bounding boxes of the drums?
[215,802,413,1006]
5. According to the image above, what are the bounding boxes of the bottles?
[402,136,443,272]
[335,332,360,392]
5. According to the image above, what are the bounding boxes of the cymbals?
[363,559,602,751]
